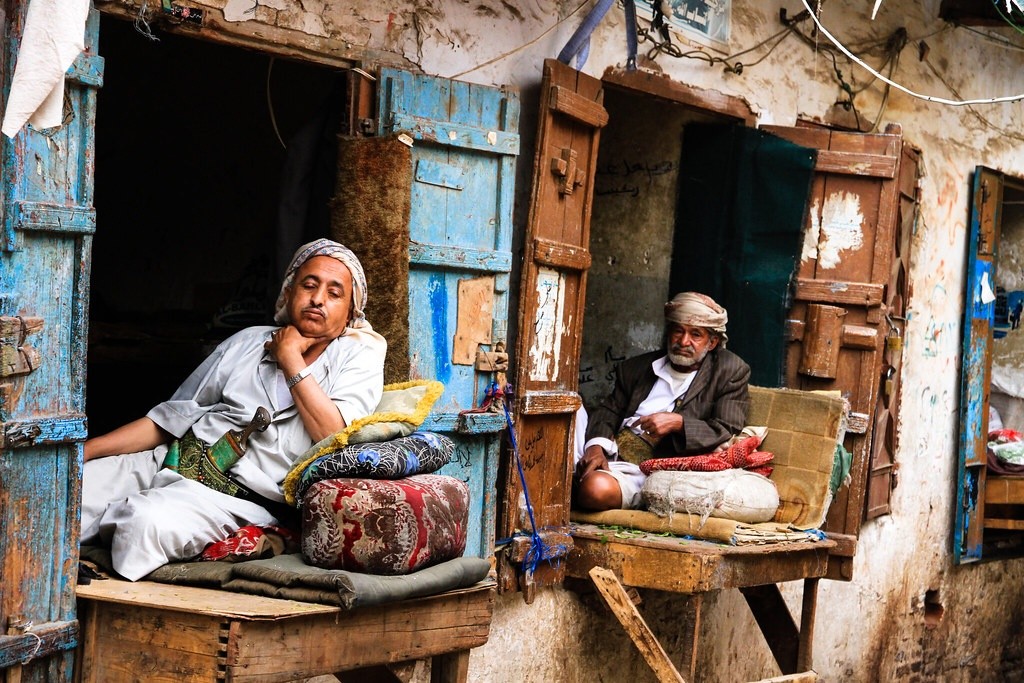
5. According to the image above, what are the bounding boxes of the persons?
[573,292,751,511]
[80,238,390,583]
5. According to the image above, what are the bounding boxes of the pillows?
[640,426,780,524]
[986,428,1024,475]
[280,378,471,576]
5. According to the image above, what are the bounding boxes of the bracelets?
[286,366,311,389]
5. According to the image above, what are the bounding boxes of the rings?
[647,431,650,434]
[271,330,277,335]
